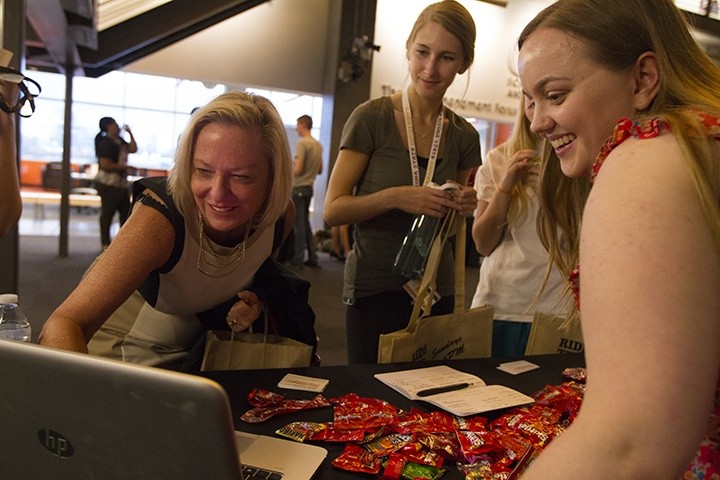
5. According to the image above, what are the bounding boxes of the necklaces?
[194,210,253,278]
[413,122,437,139]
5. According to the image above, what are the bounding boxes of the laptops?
[0,337,328,480]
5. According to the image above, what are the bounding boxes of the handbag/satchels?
[522,311,586,357]
[200,296,315,373]
[377,203,494,364]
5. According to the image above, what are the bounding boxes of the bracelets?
[496,184,513,197]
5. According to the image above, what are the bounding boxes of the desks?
[182,355,584,478]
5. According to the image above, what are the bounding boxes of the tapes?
[439,183,460,196]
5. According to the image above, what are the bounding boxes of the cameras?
[513,149,544,188]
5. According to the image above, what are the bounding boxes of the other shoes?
[303,261,319,267]
[285,262,303,270]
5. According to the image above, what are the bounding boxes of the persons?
[319,1,486,367]
[470,83,595,360]
[287,114,324,268]
[93,116,138,251]
[36,89,304,373]
[514,1,720,479]
[329,224,352,261]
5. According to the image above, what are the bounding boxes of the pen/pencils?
[416,382,475,397]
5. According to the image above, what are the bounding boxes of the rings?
[228,318,237,326]
